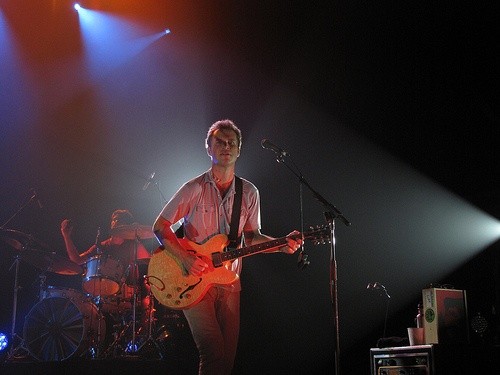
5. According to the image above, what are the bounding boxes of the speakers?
[369,344,435,375]
[423,288,474,375]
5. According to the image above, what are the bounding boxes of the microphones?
[260,138,282,156]
[95,227,102,244]
[30,187,43,208]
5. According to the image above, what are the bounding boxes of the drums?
[99,284,141,316]
[23,296,107,363]
[83,257,122,298]
[37,284,88,299]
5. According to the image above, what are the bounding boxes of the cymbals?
[111,224,155,240]
[5,228,54,251]
[42,261,81,276]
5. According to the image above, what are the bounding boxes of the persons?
[152,119,304,375]
[61,210,154,335]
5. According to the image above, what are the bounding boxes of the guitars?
[148,222,334,307]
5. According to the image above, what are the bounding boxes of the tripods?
[75,240,163,359]
